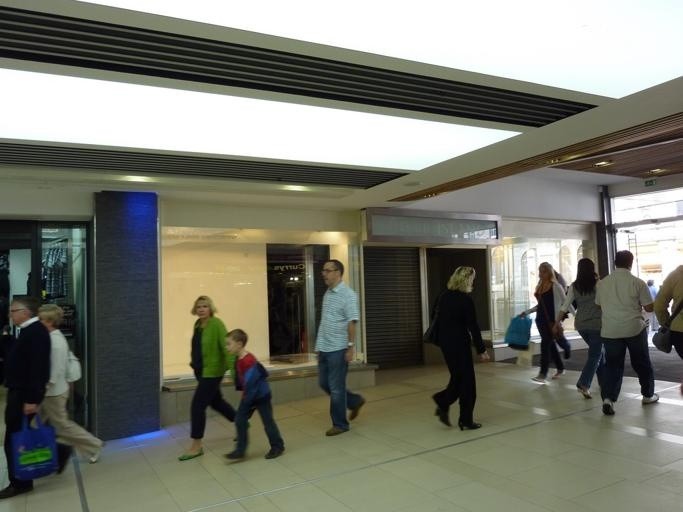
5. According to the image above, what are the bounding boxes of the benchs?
[161,361,379,393]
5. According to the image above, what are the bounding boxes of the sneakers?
[531,370,659,415]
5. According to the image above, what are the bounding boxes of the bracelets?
[347,342,354,347]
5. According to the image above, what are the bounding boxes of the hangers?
[47,238,65,252]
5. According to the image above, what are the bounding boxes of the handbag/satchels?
[65,351,82,382]
[653,326,672,353]
[11,413,59,480]
[504,315,531,350]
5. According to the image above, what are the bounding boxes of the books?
[178,295,250,460]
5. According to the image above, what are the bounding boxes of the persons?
[519,263,576,383]
[0,298,71,501]
[429,266,489,430]
[593,251,659,415]
[551,258,606,399]
[652,265,683,397]
[643,280,659,330]
[29,304,104,464]
[315,261,365,435]
[223,329,284,460]
[552,268,571,360]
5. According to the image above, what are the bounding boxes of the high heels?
[432,395,451,427]
[458,420,481,431]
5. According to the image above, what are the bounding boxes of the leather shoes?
[349,399,364,420]
[0,483,33,498]
[326,426,348,436]
[179,447,284,460]
